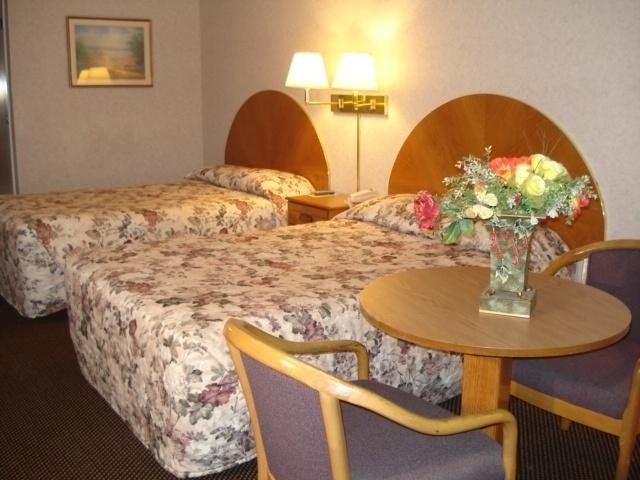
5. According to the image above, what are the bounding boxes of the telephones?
[349,188,377,204]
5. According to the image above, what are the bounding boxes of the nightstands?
[284,192,350,226]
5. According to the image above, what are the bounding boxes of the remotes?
[314,190,335,195]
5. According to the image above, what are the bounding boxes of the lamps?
[282,50,390,118]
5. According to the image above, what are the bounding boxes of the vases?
[478,212,548,320]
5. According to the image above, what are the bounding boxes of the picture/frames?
[65,15,155,89]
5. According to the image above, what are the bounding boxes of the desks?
[357,264,633,446]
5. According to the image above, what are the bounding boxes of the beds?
[63,93,607,480]
[0,88,331,322]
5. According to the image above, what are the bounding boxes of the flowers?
[411,118,602,298]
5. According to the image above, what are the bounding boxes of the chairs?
[509,238,640,480]
[221,315,519,480]
